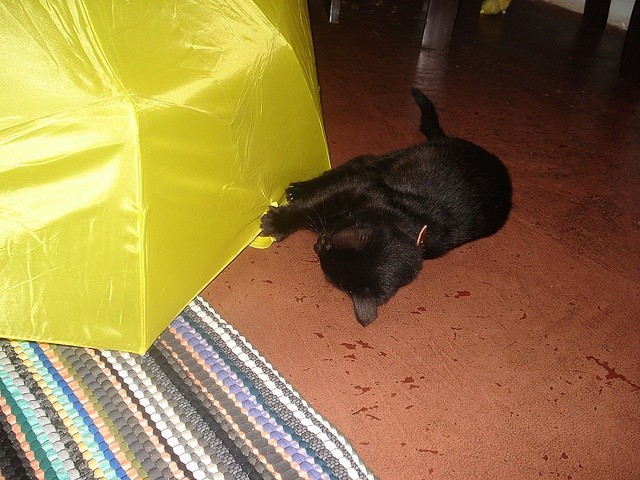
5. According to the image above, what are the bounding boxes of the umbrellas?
[0,1,332,355]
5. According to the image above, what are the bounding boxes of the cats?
[259,86,512,326]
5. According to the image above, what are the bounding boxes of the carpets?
[2,295,379,478]
